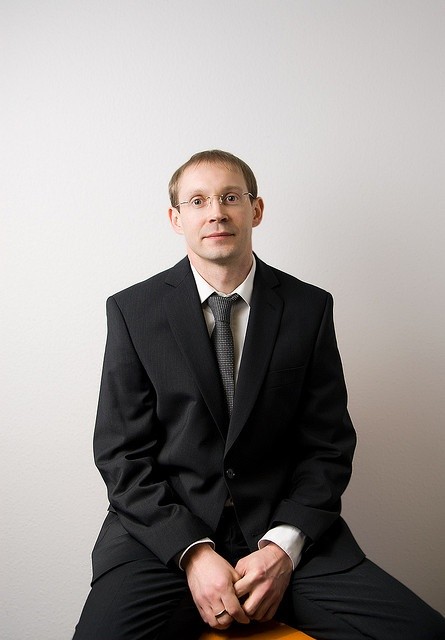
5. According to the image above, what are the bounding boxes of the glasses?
[175,192,257,208]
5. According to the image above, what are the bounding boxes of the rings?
[215,609,226,618]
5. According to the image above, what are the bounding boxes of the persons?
[72,149,444,640]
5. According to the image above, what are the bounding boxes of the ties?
[204,294,239,418]
